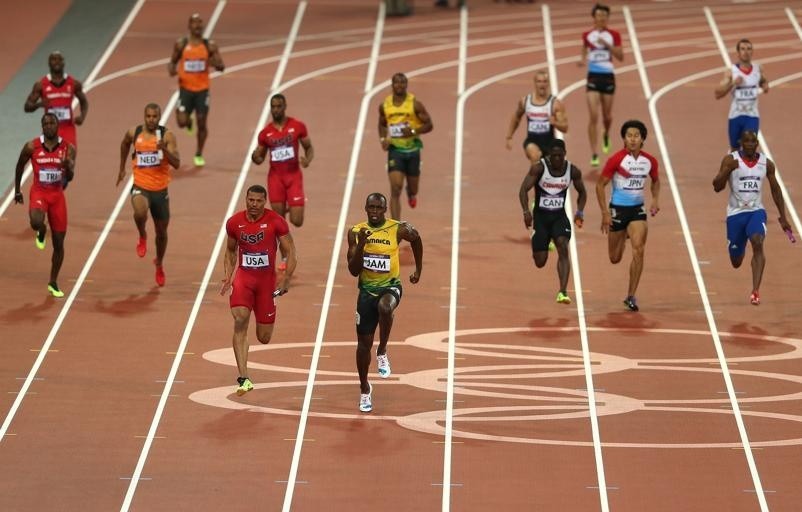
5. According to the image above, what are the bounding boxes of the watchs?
[411,128,417,136]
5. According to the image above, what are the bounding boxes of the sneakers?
[278,256,288,270]
[237,377,253,395]
[376,347,391,378]
[48,283,64,297]
[603,135,611,153]
[155,258,164,286]
[623,296,638,311]
[359,380,372,412]
[556,291,571,304]
[548,242,555,251]
[591,154,598,165]
[36,230,45,249]
[193,155,205,166]
[406,186,416,207]
[187,115,195,134]
[137,236,147,257]
[751,289,760,305]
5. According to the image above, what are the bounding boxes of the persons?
[115,102,182,286]
[24,49,88,167]
[251,93,315,272]
[714,38,770,155]
[382,1,540,19]
[502,68,570,251]
[13,112,78,299]
[575,1,626,168]
[218,183,298,397]
[518,139,588,305]
[168,12,228,167]
[710,129,793,306]
[376,71,435,222]
[344,192,424,413]
[593,119,662,312]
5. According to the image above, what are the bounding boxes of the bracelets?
[522,209,529,214]
[15,192,22,196]
[575,208,584,218]
[380,136,387,142]
